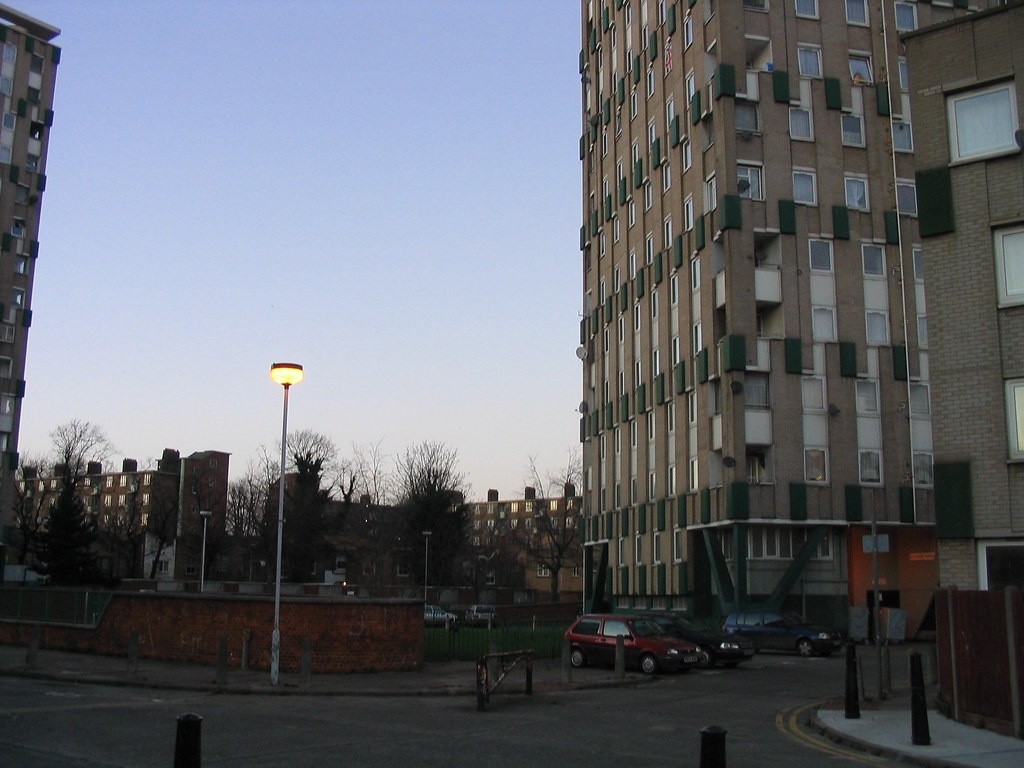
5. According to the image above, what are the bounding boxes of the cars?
[563,613,755,675]
[723,605,842,655]
[424,604,458,626]
[465,604,498,629]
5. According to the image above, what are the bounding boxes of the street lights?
[200,509,211,592]
[269,361,304,685]
[423,529,432,600]
